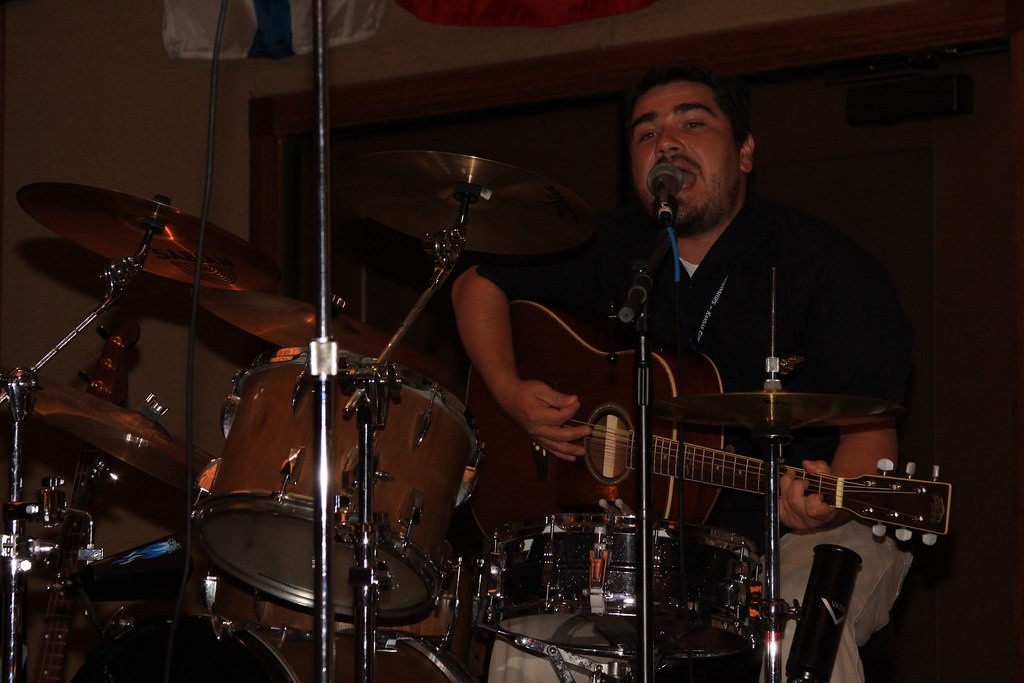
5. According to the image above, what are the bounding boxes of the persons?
[451,61,914,683]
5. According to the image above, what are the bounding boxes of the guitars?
[27,293,141,683]
[465,299,953,544]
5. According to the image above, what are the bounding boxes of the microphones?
[646,161,684,231]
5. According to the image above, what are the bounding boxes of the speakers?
[844,72,975,128]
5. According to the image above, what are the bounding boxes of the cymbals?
[339,148,595,254]
[680,388,905,426]
[16,180,276,289]
[29,376,215,490]
[196,278,418,367]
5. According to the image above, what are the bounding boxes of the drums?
[487,514,776,660]
[193,345,483,628]
[69,613,482,683]
